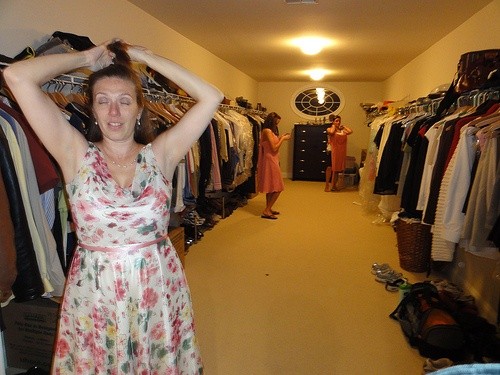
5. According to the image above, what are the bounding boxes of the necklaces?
[105,144,140,169]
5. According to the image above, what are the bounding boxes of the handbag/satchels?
[389,282,468,361]
[453,48,500,92]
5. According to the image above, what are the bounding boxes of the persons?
[322,116,353,192]
[4,39,224,375]
[256,111,291,220]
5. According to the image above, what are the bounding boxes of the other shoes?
[370,263,475,304]
[182,206,235,251]
[420,358,455,375]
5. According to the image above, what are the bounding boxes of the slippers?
[262,214,278,219]
[271,210,279,214]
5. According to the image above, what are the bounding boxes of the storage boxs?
[5,302,58,371]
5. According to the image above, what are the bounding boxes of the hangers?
[363,92,500,139]
[0,71,240,119]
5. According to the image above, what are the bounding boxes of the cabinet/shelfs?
[291,124,332,183]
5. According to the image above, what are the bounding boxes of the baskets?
[395,217,436,272]
